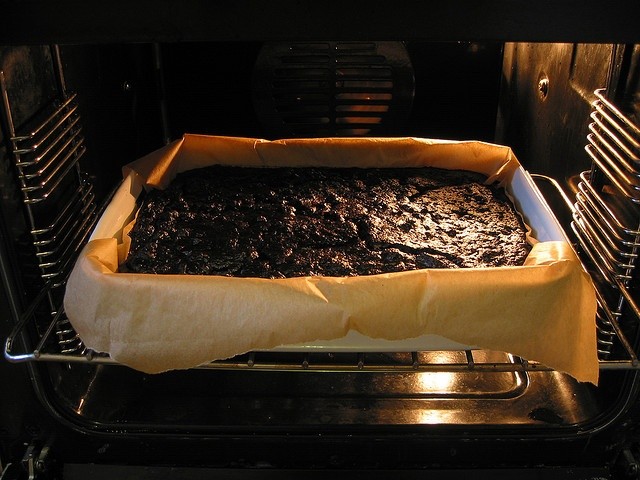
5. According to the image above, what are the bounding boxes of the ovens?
[1,1,640,480]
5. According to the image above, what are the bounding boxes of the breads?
[131,164,528,277]
